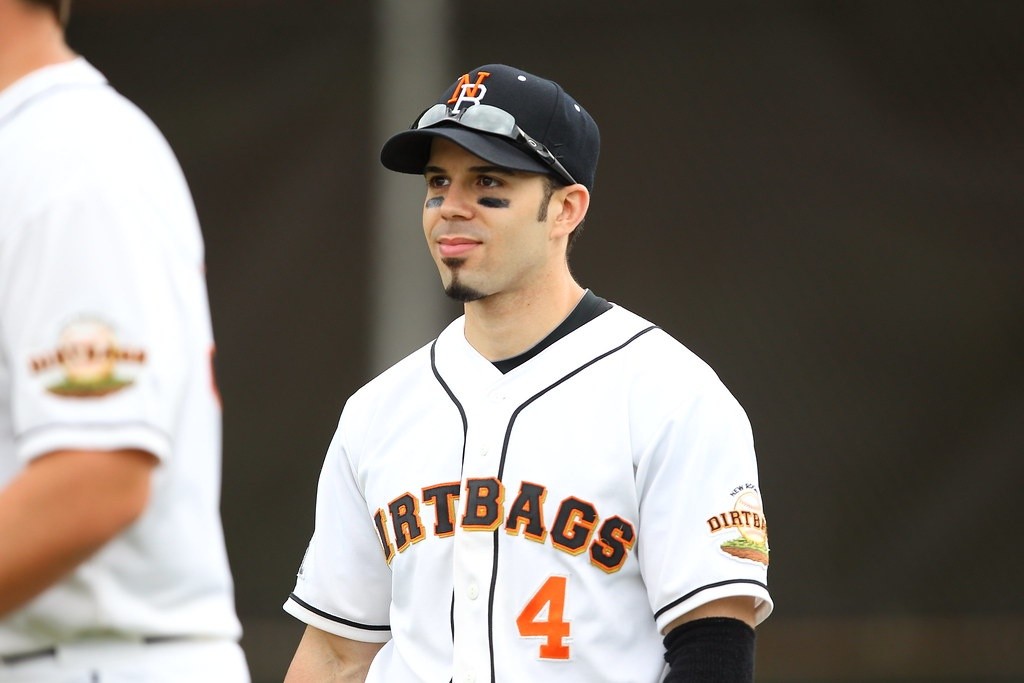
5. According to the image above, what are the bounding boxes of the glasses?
[409,104,577,186]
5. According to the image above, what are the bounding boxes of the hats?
[379,63,598,188]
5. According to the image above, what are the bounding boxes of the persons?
[0,0,253,682]
[281,61,774,683]
